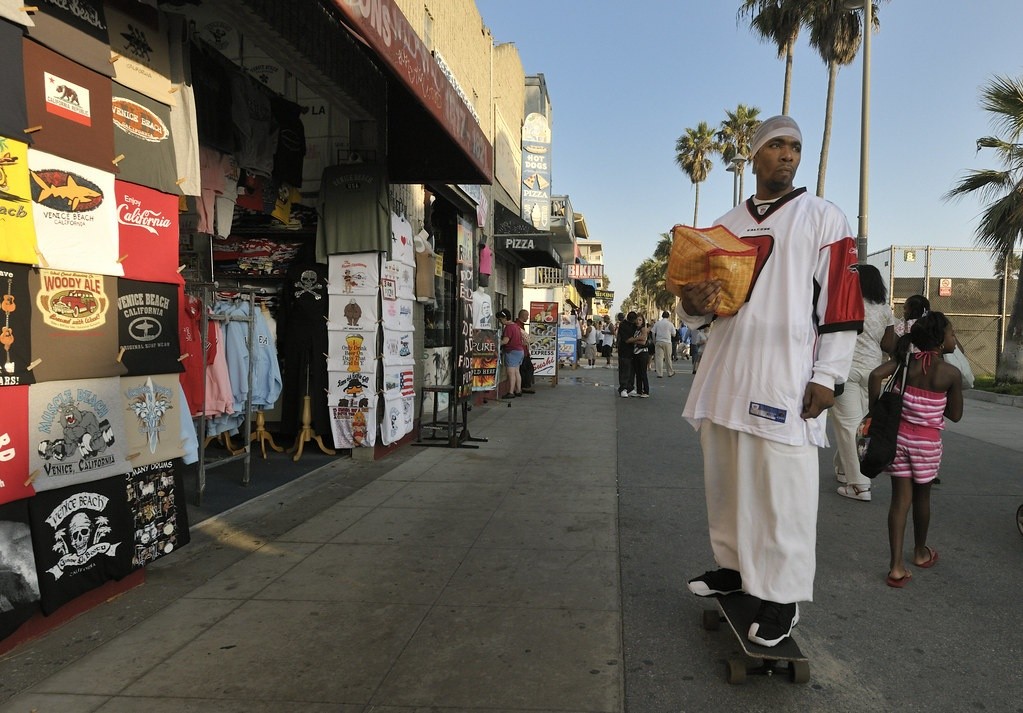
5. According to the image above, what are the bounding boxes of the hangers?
[182,284,203,304]
[345,151,363,165]
[216,290,252,310]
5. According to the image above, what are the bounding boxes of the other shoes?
[592,364,596,368]
[657,375,663,378]
[502,392,515,399]
[602,364,611,369]
[633,394,649,397]
[514,390,522,397]
[669,371,675,377]
[521,389,535,394]
[583,364,592,369]
[620,389,628,398]
[628,389,637,396]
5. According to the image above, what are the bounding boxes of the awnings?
[576,257,596,297]
[494,236,563,270]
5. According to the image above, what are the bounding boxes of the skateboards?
[702,570,811,684]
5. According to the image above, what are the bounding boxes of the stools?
[417,386,465,440]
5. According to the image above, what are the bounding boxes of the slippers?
[918,546,939,567]
[887,568,912,587]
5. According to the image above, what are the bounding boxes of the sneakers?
[687,568,744,597]
[838,486,872,500]
[747,602,800,648]
[835,467,847,483]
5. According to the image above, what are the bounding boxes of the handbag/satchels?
[520,328,530,346]
[648,343,656,354]
[855,351,909,480]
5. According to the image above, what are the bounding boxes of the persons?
[678,115,863,647]
[671,322,709,374]
[479,241,493,287]
[868,313,963,589]
[601,315,615,369]
[496,309,525,399]
[472,287,494,329]
[570,310,584,366]
[593,313,626,357]
[647,311,676,378]
[413,229,437,304]
[831,265,895,501]
[583,319,597,369]
[514,310,534,395]
[895,296,941,484]
[617,311,649,398]
